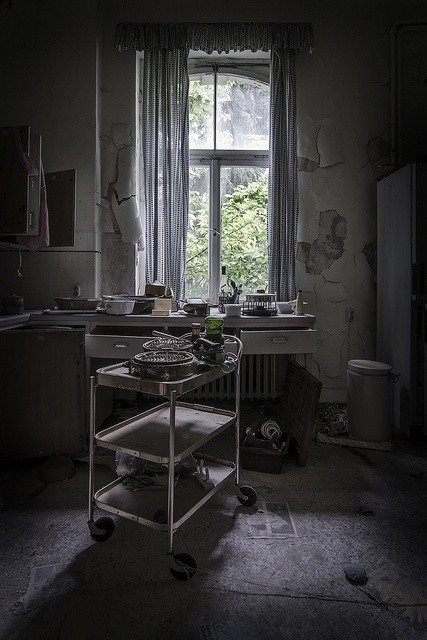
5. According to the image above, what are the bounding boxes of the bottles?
[295,289,304,313]
[218,267,227,313]
[190,322,201,348]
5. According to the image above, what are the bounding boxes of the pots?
[53,283,103,310]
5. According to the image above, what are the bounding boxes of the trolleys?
[87,331,256,582]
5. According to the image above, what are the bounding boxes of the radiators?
[140,355,275,399]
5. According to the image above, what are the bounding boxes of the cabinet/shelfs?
[1,125,77,255]
[0,325,88,462]
[85,312,318,358]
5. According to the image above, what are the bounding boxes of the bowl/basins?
[224,302,243,317]
[103,298,137,316]
